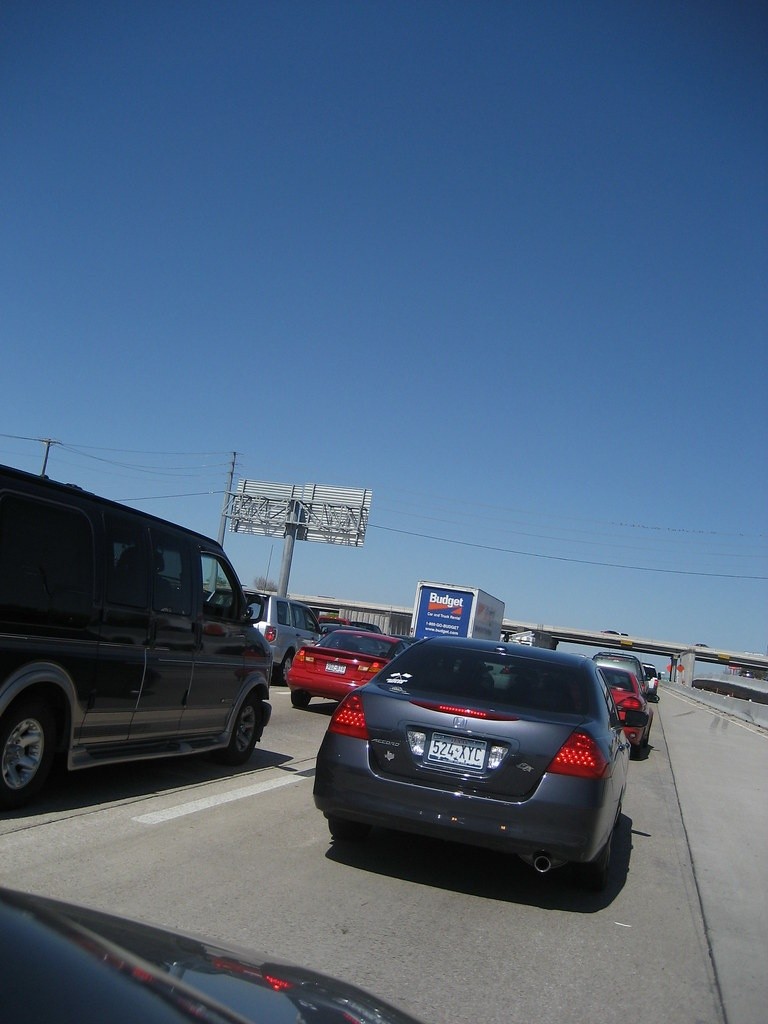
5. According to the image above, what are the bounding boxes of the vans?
[208,581,327,687]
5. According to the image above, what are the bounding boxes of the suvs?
[1,461,271,814]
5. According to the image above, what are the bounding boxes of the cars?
[601,630,629,638]
[594,660,654,761]
[284,628,421,715]
[0,888,424,1024]
[311,635,651,885]
[314,578,661,707]
[695,644,709,648]
